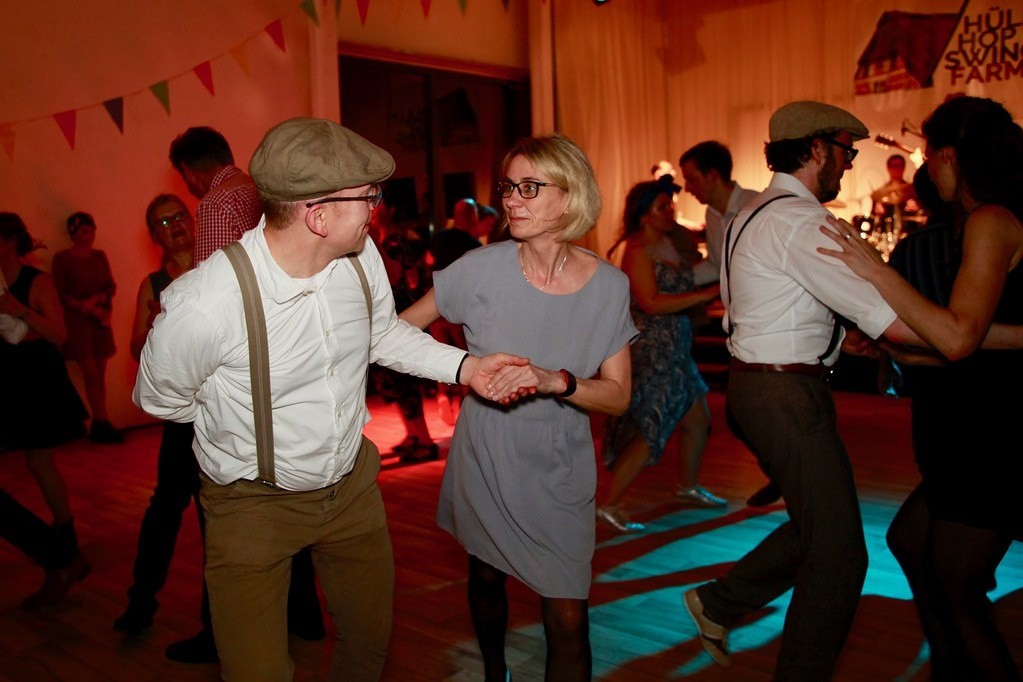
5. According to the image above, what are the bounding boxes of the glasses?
[154,210,188,227]
[498,178,568,200]
[304,184,383,210]
[823,137,859,163]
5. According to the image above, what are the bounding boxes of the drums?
[852,214,875,237]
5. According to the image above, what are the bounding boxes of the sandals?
[400,444,439,465]
[392,435,416,454]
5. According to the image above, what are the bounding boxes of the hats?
[247,117,396,201]
[769,103,870,142]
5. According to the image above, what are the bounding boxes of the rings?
[845,235,850,239]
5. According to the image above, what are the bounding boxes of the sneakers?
[684,589,732,669]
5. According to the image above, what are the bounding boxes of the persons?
[477,204,498,237]
[0,213,94,609]
[50,213,124,445]
[371,194,439,465]
[817,96,1023,682]
[114,195,198,636]
[429,198,484,425]
[165,126,326,664]
[595,180,728,531]
[683,100,1023,682]
[871,155,919,233]
[397,133,641,682]
[679,140,783,507]
[132,117,537,682]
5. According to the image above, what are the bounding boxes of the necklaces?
[520,254,569,290]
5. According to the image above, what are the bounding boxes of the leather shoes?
[674,483,729,505]
[597,508,645,532]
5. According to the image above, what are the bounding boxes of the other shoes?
[285,613,325,639]
[116,609,154,630]
[21,555,90,609]
[747,480,782,507]
[89,419,124,440]
[166,630,220,662]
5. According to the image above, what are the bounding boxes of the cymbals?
[871,183,915,204]
[822,199,847,207]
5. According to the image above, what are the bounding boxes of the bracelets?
[18,308,32,319]
[559,369,576,397]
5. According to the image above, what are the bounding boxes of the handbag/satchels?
[0,338,89,449]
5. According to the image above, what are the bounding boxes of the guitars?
[872,131,928,162]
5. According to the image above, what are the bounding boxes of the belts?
[731,357,829,374]
